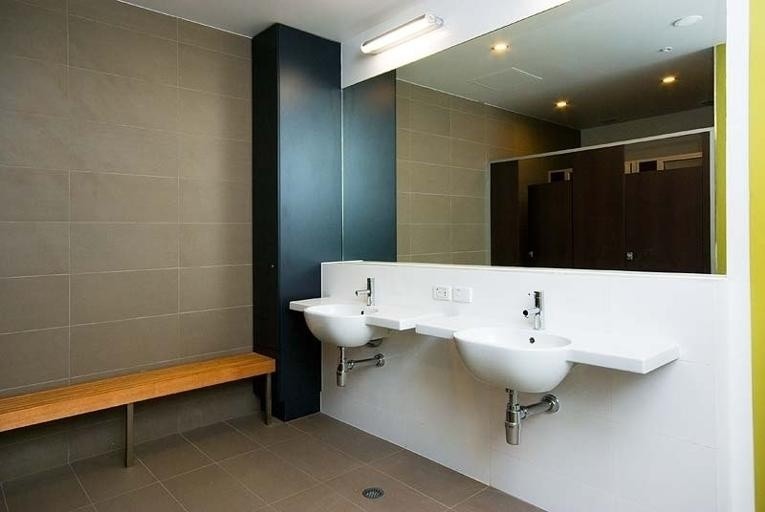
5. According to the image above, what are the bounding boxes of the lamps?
[360,14,442,56]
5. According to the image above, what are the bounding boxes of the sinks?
[452,329,572,393]
[304,304,377,347]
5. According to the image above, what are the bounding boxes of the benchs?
[0,351,277,468]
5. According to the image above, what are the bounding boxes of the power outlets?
[432,285,451,301]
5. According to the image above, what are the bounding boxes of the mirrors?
[342,1,728,276]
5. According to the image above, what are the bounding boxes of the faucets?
[355,277,375,306]
[522,291,544,330]
